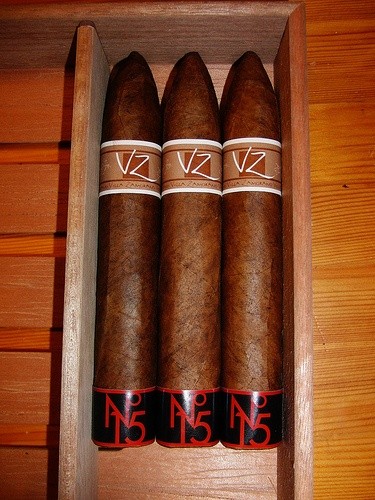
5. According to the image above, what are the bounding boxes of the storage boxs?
[4,0,310,500]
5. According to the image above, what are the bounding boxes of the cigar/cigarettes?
[94,50,158,452]
[157,48,219,449]
[215,48,286,451]
